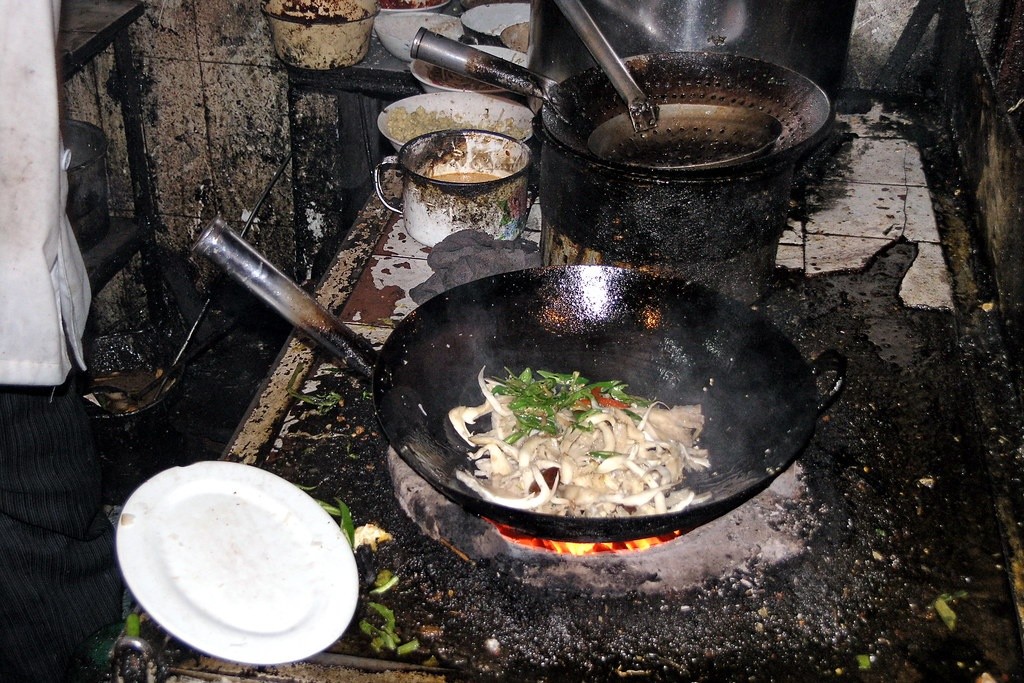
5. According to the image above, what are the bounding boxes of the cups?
[372,130,535,248]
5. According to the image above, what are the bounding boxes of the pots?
[57,118,112,250]
[408,29,835,178]
[195,214,853,539]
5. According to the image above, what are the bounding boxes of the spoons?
[78,147,297,420]
[559,0,782,168]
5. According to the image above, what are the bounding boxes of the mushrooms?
[448,365,710,517]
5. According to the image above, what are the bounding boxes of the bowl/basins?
[260,0,530,70]
[377,91,536,156]
[411,45,532,98]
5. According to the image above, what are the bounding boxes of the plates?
[114,458,360,666]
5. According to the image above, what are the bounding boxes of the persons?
[2,0,136,680]
